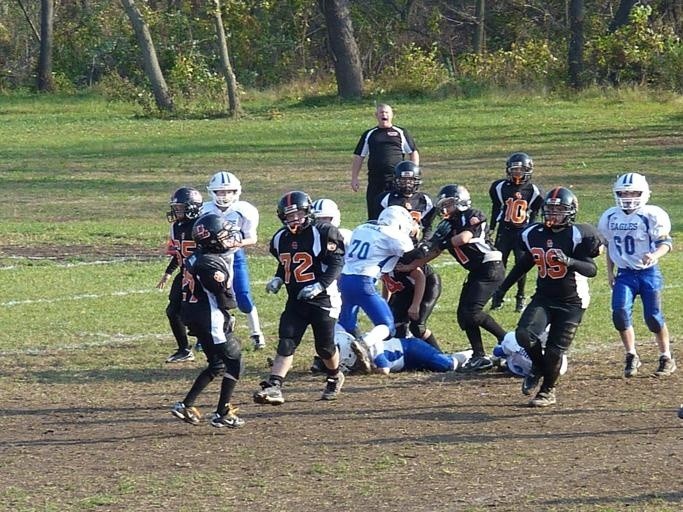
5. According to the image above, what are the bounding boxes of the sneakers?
[310,354,325,372]
[624,351,641,378]
[172,400,202,425]
[210,407,245,428]
[455,350,492,373]
[529,385,556,406]
[250,332,267,350]
[655,356,677,377]
[253,382,285,405]
[321,371,346,400]
[522,370,541,395]
[167,347,196,363]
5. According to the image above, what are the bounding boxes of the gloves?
[432,221,452,243]
[551,249,570,266]
[491,289,505,309]
[265,277,284,294]
[298,282,324,301]
[222,311,238,334]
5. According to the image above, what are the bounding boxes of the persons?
[193,169,266,353]
[597,171,678,378]
[310,159,506,372]
[155,184,207,362]
[250,192,353,405]
[170,214,247,430]
[350,103,418,222]
[484,150,544,313]
[489,186,604,407]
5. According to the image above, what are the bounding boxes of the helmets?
[614,173,650,211]
[392,160,423,196]
[278,191,341,232]
[334,331,357,373]
[376,204,413,235]
[505,153,534,183]
[170,172,244,253]
[435,184,470,219]
[542,187,579,228]
